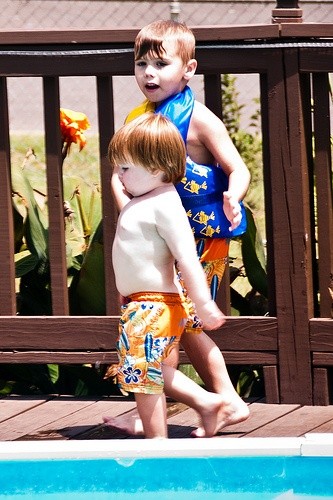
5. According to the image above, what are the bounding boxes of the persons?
[104,19,251,438]
[108,109,225,439]
